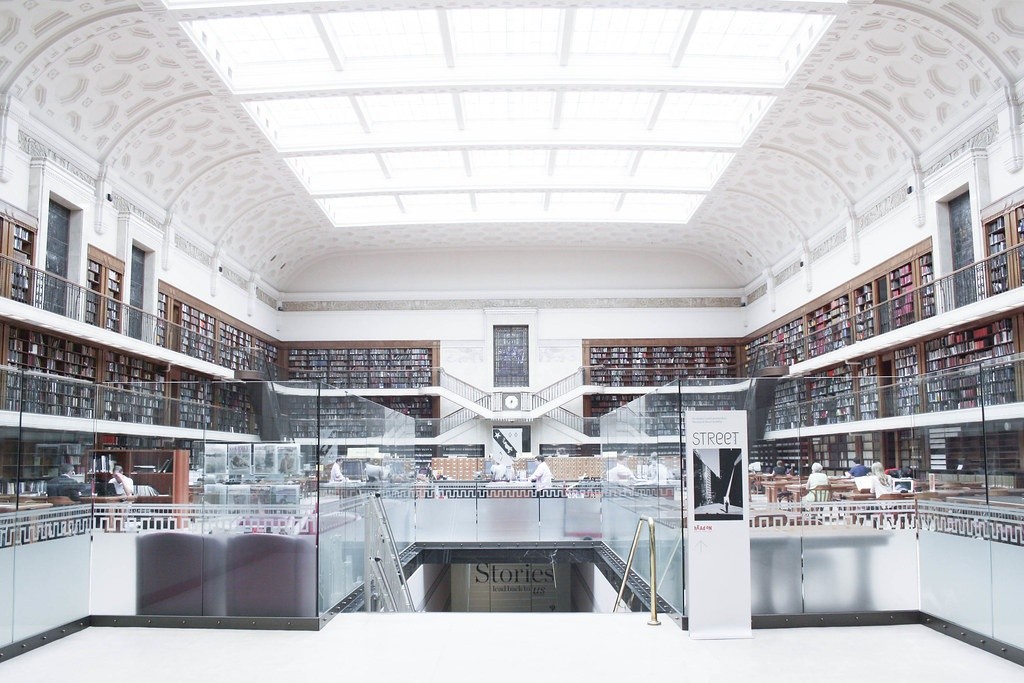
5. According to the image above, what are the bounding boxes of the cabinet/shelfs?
[581,202,1024,474]
[0,213,440,505]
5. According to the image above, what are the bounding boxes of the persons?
[899,468,913,478]
[645,452,657,481]
[108,465,133,496]
[609,454,646,482]
[527,455,555,489]
[870,461,893,510]
[331,458,349,483]
[802,462,832,519]
[845,457,872,477]
[47,463,80,502]
[766,460,788,477]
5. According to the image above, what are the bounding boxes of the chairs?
[807,485,832,502]
[47,497,83,507]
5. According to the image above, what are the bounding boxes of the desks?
[749,474,1024,508]
[0,496,130,545]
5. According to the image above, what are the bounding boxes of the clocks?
[505,394,519,409]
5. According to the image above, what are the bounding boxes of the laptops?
[95,482,122,497]
[79,483,92,497]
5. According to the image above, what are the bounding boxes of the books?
[0,225,484,495]
[541,206,1024,489]
